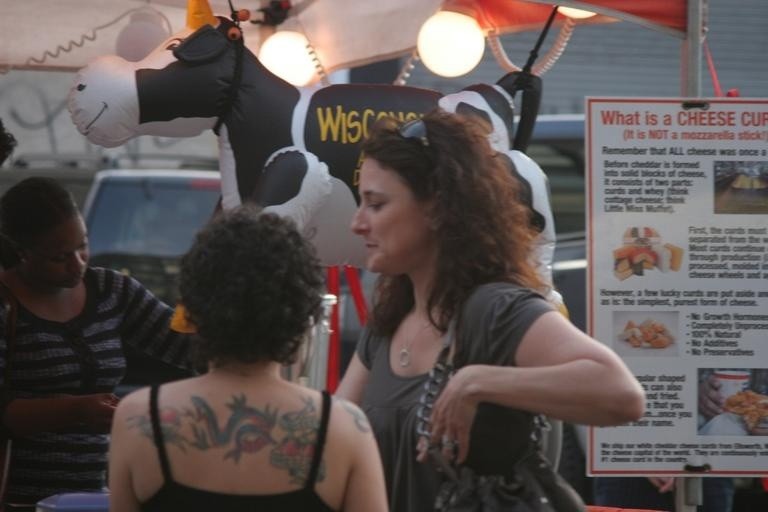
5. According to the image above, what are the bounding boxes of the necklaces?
[397,318,426,367]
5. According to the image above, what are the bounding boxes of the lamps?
[258,1,321,89]
[414,0,486,76]
[554,0,595,18]
[115,0,173,63]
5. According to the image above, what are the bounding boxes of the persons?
[328,104,647,512]
[108,204,391,511]
[1,174,208,512]
[576,424,733,511]
[697,371,767,436]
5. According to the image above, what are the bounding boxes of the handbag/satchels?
[429,451,589,512]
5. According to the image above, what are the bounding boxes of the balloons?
[68,0,570,317]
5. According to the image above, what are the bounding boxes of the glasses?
[396,118,443,174]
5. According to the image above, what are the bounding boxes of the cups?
[709,370,752,411]
